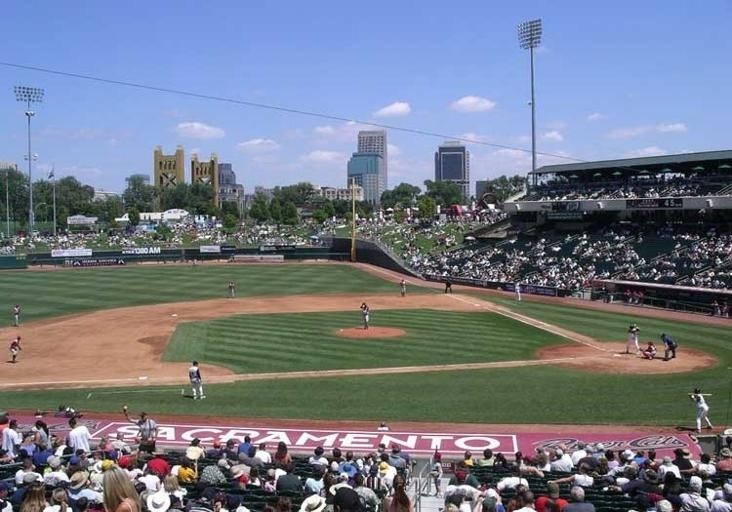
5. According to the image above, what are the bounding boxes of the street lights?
[517,17,542,185]
[13,85,45,227]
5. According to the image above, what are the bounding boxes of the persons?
[1,228,98,256]
[400,279,407,297]
[106,214,235,247]
[235,212,377,246]
[9,337,23,364]
[229,280,235,297]
[522,170,731,317]
[1,405,732,511]
[514,282,521,301]
[360,302,369,328]
[641,343,656,360]
[376,209,529,283]
[659,334,678,363]
[11,304,21,327]
[187,360,206,400]
[689,388,714,432]
[626,324,640,357]
[445,279,453,294]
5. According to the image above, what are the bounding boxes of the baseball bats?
[688,393,712,396]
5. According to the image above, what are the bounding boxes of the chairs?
[161,447,684,511]
